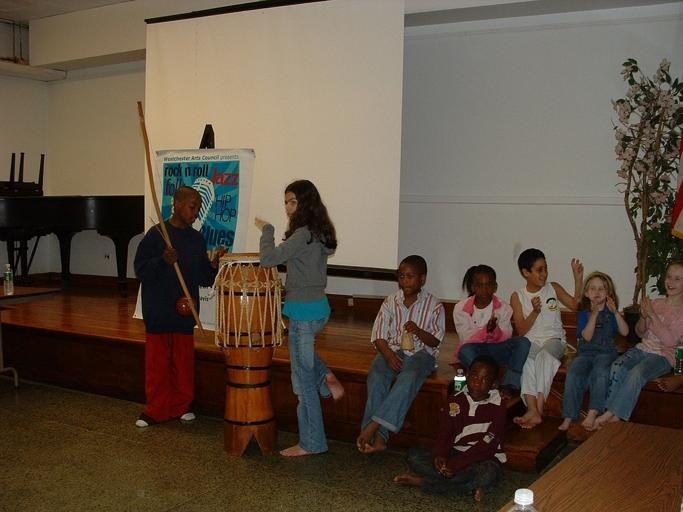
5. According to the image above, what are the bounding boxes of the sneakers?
[179,411,195,420]
[135,415,154,427]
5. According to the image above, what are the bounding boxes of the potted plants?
[608,58,683,344]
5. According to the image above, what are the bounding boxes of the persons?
[356,255,446,454]
[255,180,345,456]
[656,375,683,392]
[594,262,683,428]
[511,248,583,429]
[394,355,510,501]
[559,271,629,430]
[454,265,531,398]
[134,185,229,428]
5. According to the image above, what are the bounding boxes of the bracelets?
[642,317,647,320]
[613,309,618,314]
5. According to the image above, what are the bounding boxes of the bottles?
[673,335,683,375]
[2,263,12,296]
[452,368,464,394]
[507,489,538,512]
[400,327,415,350]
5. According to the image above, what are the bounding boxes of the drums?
[215,253,285,458]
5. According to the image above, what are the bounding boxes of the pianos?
[0,196,144,298]
